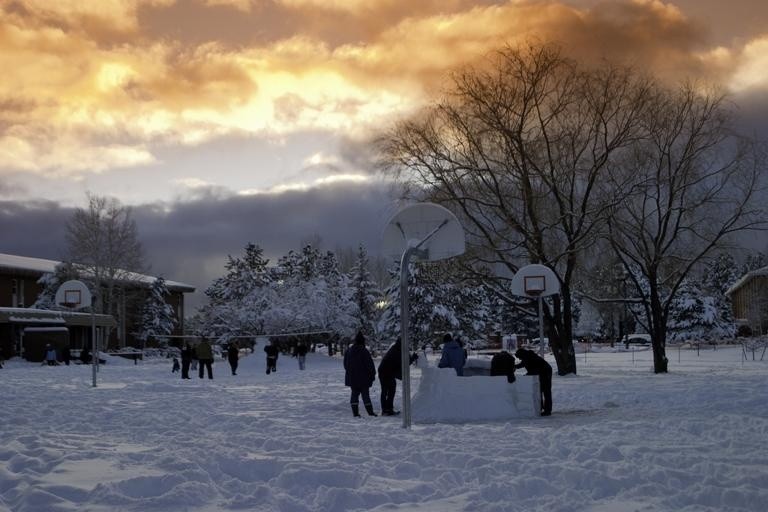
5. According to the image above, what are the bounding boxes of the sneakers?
[542,411,551,416]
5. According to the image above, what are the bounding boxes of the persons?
[490,349,552,416]
[343,332,419,417]
[172,338,308,379]
[46,346,56,366]
[439,334,465,376]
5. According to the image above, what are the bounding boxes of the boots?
[382,408,401,416]
[351,403,360,417]
[364,402,378,417]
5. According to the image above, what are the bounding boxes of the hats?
[515,349,524,358]
[443,334,452,343]
[355,331,366,344]
